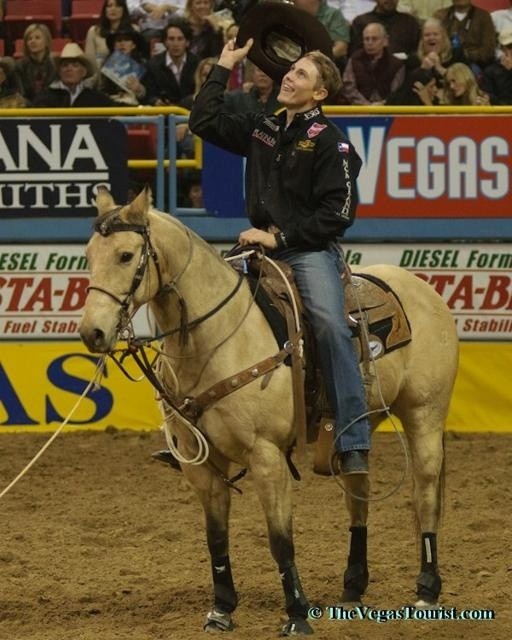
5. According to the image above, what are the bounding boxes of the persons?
[481,43,512,106]
[350,0,421,55]
[343,22,406,107]
[95,20,155,107]
[128,7,147,34]
[249,63,277,102]
[140,19,199,107]
[180,56,229,110]
[85,0,141,71]
[33,43,116,108]
[0,56,32,109]
[220,23,251,94]
[443,62,491,106]
[383,68,438,106]
[293,0,352,60]
[433,0,498,66]
[407,17,463,90]
[188,36,374,476]
[184,0,213,56]
[12,23,60,104]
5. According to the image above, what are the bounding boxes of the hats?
[52,41,95,80]
[499,27,511,46]
[232,1,336,86]
[105,20,145,54]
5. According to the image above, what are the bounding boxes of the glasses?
[362,35,386,44]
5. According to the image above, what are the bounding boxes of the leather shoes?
[337,450,369,474]
[150,448,180,465]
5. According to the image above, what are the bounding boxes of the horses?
[77,181,459,640]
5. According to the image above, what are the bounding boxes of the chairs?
[1,2,60,39]
[473,0,512,14]
[125,123,158,184]
[63,2,107,42]
[13,39,72,58]
